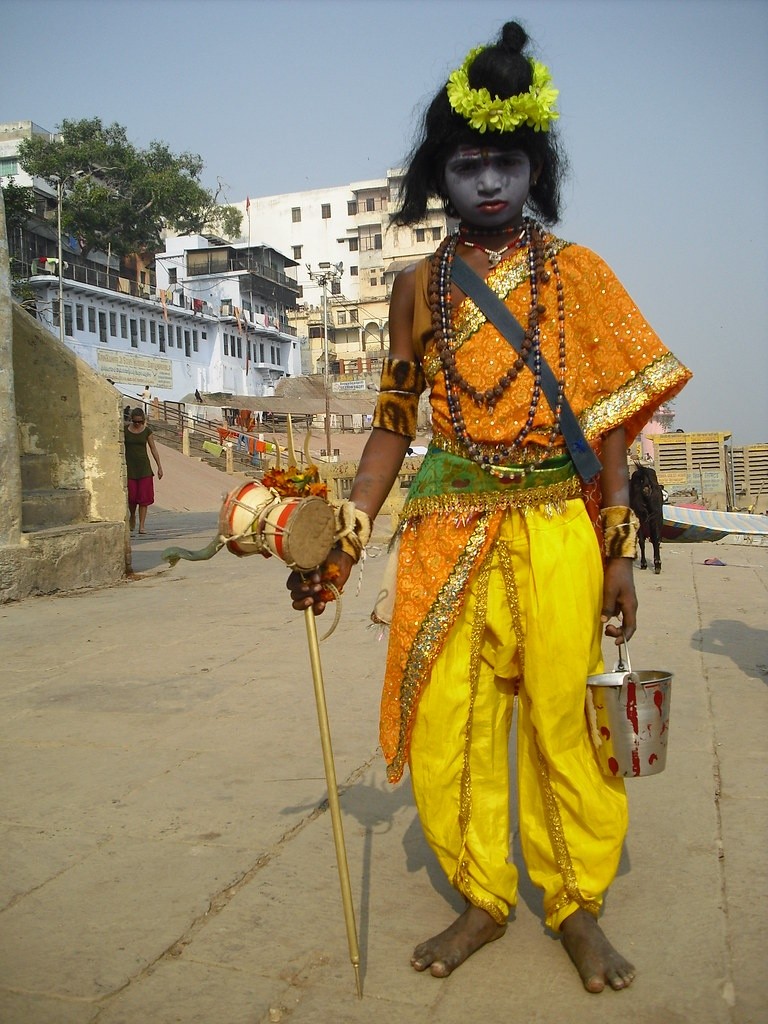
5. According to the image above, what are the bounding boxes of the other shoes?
[129,516,135,531]
[139,529,147,534]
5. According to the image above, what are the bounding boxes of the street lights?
[305,262,344,457]
[47,170,86,341]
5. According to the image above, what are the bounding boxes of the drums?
[217,476,338,574]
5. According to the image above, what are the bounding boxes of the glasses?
[132,419,144,423]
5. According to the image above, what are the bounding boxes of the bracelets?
[330,498,374,563]
[600,506,641,560]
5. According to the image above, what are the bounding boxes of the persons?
[124,405,163,534]
[195,391,200,402]
[659,485,669,505]
[138,386,151,419]
[748,502,768,516]
[286,21,693,994]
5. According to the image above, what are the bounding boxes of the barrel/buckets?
[586,626,675,778]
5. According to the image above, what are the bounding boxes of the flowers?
[446,45,559,135]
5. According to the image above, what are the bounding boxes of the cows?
[629,461,665,574]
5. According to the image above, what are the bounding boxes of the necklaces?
[428,216,567,479]
